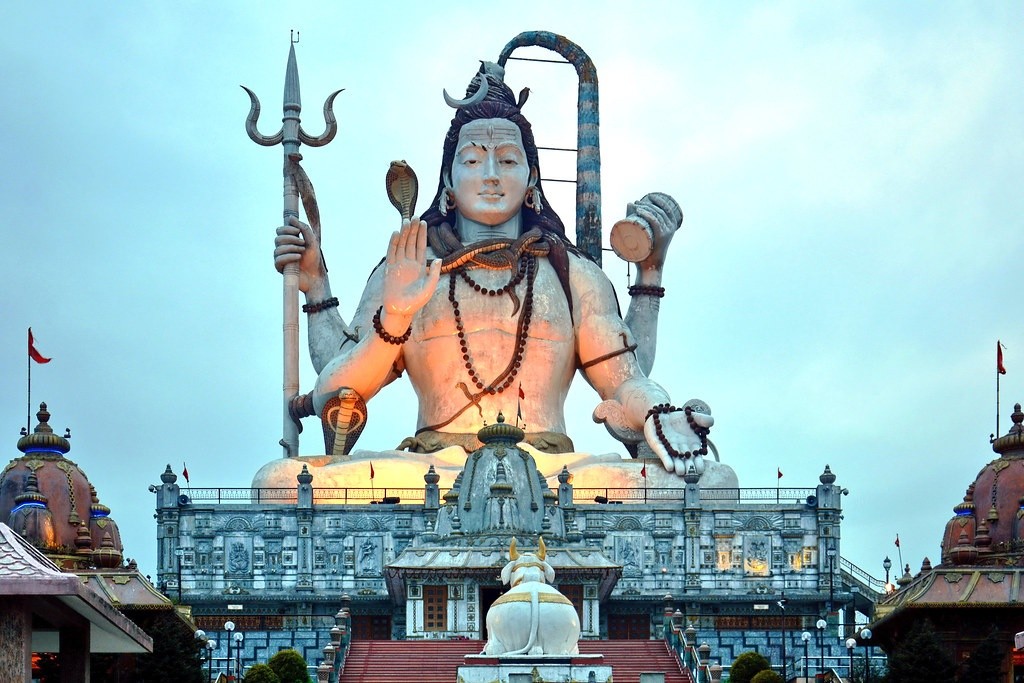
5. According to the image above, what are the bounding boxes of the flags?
[28,327,52,365]
[894,534,899,547]
[519,382,524,400]
[518,402,522,420]
[641,458,646,477]
[997,341,1007,375]
[183,462,189,482]
[369,460,375,479]
[778,467,783,478]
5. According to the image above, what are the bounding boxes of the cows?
[479,535,580,656]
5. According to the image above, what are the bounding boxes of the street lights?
[206,640,217,683]
[846,638,856,683]
[816,620,827,674]
[175,546,184,602]
[194,629,205,683]
[883,555,892,595]
[224,621,235,683]
[861,629,873,683]
[234,633,243,683]
[801,632,812,683]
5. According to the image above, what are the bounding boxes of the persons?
[251,60,741,506]
